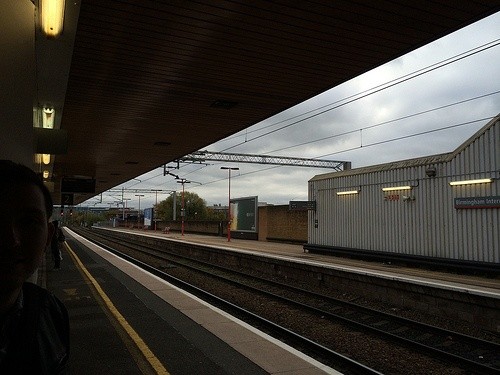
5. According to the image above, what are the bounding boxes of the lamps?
[336,190,360,196]
[381,185,413,191]
[43,154,50,165]
[42,107,55,129]
[42,170,50,179]
[40,0,66,39]
[448,178,494,187]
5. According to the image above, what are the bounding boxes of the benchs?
[161,226,170,234]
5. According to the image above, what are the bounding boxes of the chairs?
[142,225,148,232]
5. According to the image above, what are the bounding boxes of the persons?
[51,219,65,268]
[0,159,71,375]
